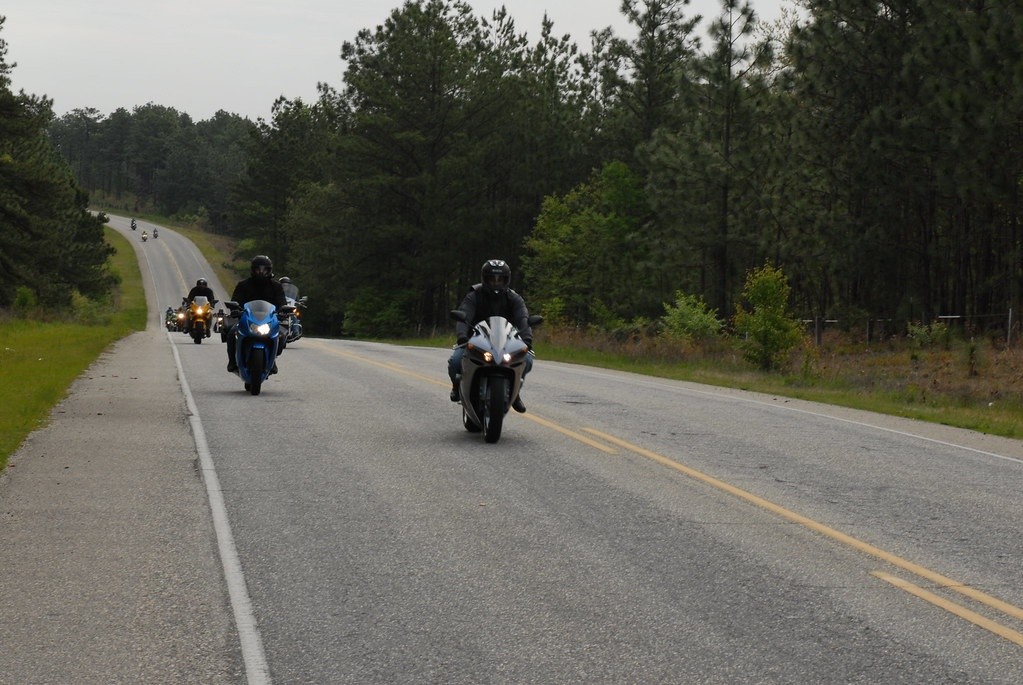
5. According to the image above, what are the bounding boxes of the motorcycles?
[449,310,544,443]
[132,221,159,242]
[223,300,297,396]
[166,282,308,355]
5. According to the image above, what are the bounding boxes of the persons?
[154,228,158,234]
[172,308,178,316]
[166,307,173,315]
[143,231,147,235]
[225,255,287,374]
[213,309,226,330]
[279,277,299,337]
[183,278,215,337]
[448,259,533,413]
[131,218,135,224]
[178,301,186,312]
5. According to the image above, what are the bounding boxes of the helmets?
[250,255,273,277]
[278,277,290,283]
[481,260,511,295]
[219,308,223,314]
[197,278,207,287]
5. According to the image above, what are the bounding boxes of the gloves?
[457,336,468,349]
[523,339,532,351]
[231,310,240,319]
[211,305,215,308]
[277,305,294,314]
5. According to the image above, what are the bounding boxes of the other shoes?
[227,358,237,372]
[206,328,210,338]
[269,362,278,374]
[450,384,461,401]
[183,327,189,334]
[292,332,299,338]
[509,390,526,413]
